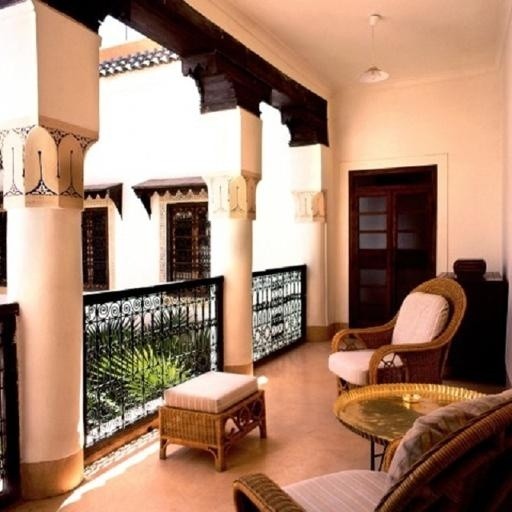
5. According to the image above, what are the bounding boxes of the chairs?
[231,387,512,512]
[326,277,468,398]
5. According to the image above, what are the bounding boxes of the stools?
[159,371,268,472]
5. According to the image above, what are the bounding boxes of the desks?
[329,380,489,473]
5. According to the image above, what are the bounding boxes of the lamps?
[356,14,393,84]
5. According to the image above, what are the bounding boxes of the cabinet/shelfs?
[437,271,509,387]
